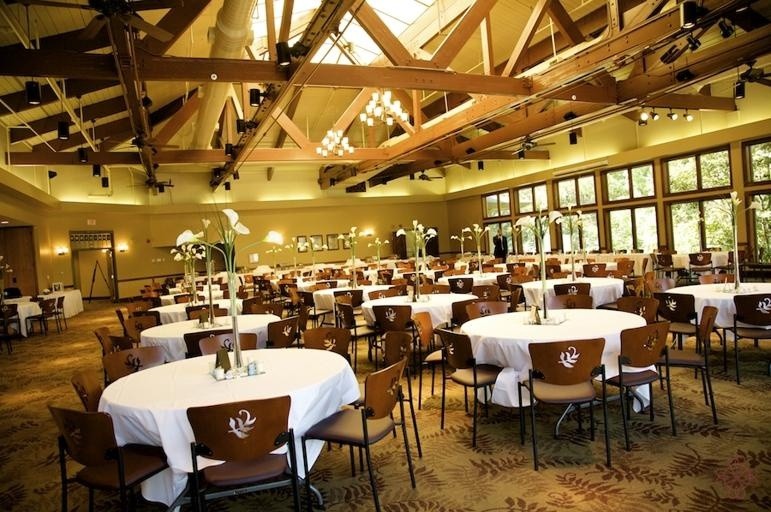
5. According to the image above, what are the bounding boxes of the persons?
[493,229,509,263]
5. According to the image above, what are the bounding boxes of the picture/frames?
[327,233,340,251]
[342,232,353,250]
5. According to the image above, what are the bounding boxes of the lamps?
[717,18,734,39]
[416,130,580,181]
[684,30,702,52]
[130,90,167,195]
[649,106,659,122]
[637,108,647,127]
[21,77,109,192]
[682,109,696,123]
[359,90,409,128]
[208,41,305,192]
[315,127,358,161]
[667,108,679,121]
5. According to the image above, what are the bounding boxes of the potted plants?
[560,204,586,243]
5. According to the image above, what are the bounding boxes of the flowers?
[339,226,374,256]
[176,207,286,297]
[420,226,438,251]
[463,222,491,249]
[513,201,565,265]
[389,219,434,260]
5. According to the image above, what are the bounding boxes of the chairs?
[0,238,771,510]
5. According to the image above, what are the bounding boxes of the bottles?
[716,280,756,293]
[214,356,260,380]
